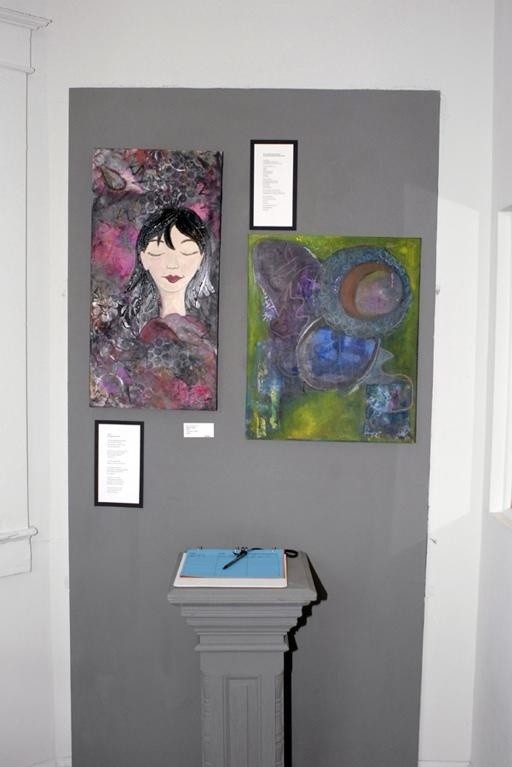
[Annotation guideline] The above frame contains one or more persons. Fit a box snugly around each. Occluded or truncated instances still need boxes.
[114,204,217,402]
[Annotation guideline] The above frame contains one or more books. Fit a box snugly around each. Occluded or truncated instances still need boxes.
[179,544,285,581]
[173,550,288,589]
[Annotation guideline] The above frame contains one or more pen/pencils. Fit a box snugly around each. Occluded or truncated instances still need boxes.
[223,551,247,569]
